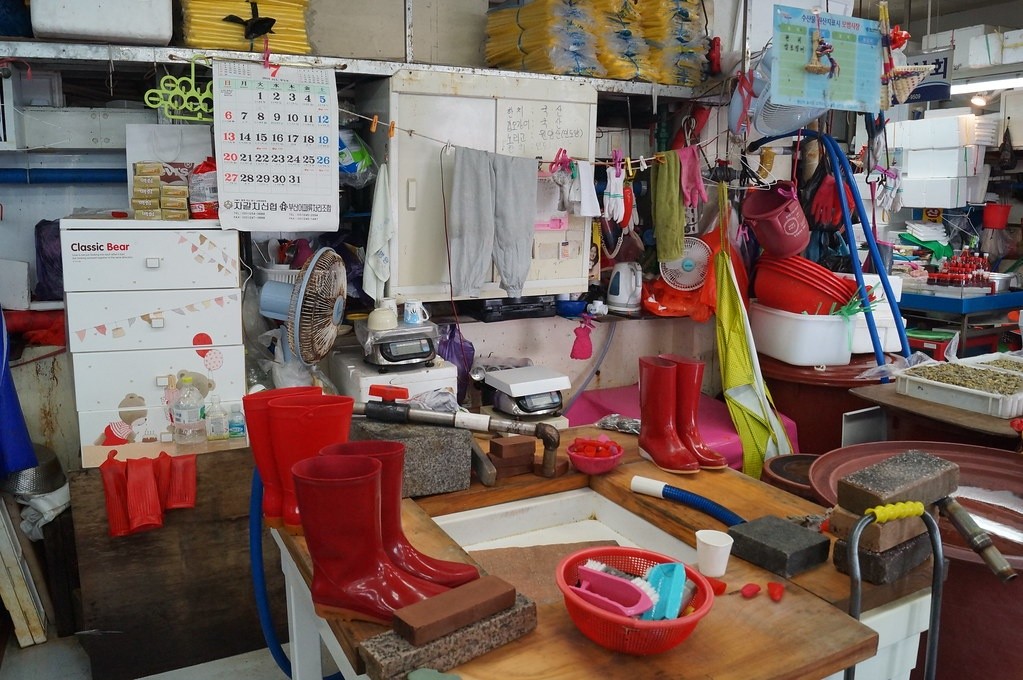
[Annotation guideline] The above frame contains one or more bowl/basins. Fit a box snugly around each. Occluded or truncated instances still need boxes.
[368,307,397,330]
[755,250,854,315]
[555,300,587,317]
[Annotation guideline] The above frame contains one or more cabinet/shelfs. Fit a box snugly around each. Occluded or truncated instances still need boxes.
[356,71,599,305]
[997,89,1023,147]
[0,59,176,152]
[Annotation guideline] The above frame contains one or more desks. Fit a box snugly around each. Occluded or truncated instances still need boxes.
[849,382,1023,442]
[266,425,948,680]
[896,292,1023,359]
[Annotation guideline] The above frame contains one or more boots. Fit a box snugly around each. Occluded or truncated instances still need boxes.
[266,393,354,535]
[659,354,728,470]
[242,386,325,530]
[638,356,699,473]
[320,439,479,587]
[293,455,450,627]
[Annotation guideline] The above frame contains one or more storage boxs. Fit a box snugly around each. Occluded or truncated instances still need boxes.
[30,0,174,48]
[59,163,250,466]
[327,348,459,404]
[749,266,1022,421]
[846,102,987,250]
[920,23,1023,69]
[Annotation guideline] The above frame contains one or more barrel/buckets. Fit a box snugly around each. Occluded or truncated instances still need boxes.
[742,180,810,259]
[983,203,1013,230]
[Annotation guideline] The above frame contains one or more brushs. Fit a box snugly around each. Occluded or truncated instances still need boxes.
[568,558,660,616]
[637,562,686,620]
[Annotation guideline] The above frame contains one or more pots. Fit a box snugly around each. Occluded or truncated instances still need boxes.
[989,273,1019,293]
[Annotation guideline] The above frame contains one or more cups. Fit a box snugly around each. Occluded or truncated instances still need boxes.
[404,299,429,325]
[380,298,398,317]
[695,530,734,577]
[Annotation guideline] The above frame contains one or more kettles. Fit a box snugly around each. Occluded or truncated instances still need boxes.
[605,261,642,312]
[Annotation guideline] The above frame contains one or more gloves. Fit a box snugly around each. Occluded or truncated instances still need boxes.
[609,169,625,223]
[675,143,708,207]
[99,448,197,538]
[623,181,639,234]
[811,174,855,224]
[603,169,613,222]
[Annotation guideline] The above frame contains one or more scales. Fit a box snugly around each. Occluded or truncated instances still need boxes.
[354,317,441,374]
[484,365,572,421]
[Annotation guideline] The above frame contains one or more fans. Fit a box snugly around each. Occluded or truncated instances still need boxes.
[728,47,830,138]
[258,247,348,395]
[659,236,713,292]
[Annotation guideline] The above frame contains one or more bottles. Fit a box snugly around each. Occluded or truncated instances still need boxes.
[926,246,990,288]
[229,404,246,438]
[173,376,206,444]
[205,395,230,441]
[990,281,996,294]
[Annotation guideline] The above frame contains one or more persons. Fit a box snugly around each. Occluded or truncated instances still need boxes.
[591,241,600,274]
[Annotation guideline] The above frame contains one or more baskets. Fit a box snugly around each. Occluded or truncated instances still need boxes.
[558,546,715,656]
[565,440,624,475]
